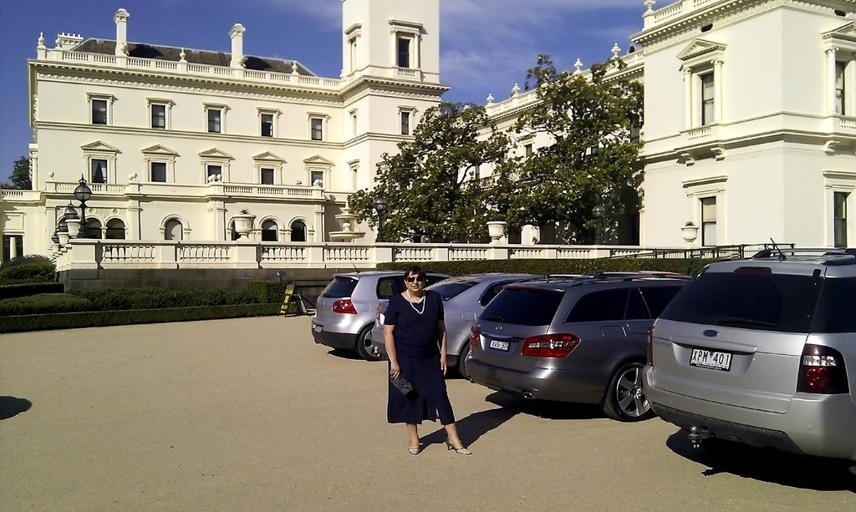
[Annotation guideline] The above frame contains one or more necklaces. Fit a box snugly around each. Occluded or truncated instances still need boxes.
[406,289,426,316]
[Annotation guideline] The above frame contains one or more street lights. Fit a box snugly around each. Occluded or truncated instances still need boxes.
[50,233,58,243]
[57,217,67,231]
[73,173,96,238]
[63,200,80,219]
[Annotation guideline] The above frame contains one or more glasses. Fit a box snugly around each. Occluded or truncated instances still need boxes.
[407,276,424,282]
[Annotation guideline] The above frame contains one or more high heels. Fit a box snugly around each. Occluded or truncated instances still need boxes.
[446,439,472,455]
[408,435,420,455]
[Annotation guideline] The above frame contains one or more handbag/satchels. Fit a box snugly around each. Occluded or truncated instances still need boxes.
[390,373,413,396]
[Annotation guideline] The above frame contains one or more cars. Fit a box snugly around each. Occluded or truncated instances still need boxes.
[311,270,453,361]
[370,270,551,380]
[641,248,856,465]
[464,269,691,423]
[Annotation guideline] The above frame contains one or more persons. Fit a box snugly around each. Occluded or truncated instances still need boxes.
[381,265,472,455]
